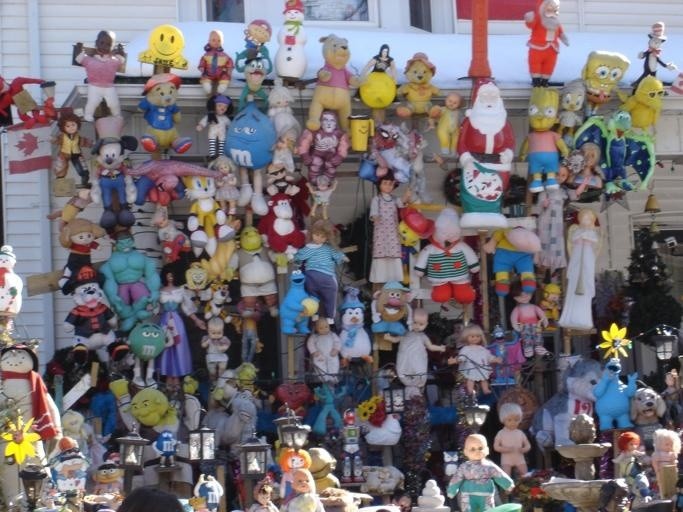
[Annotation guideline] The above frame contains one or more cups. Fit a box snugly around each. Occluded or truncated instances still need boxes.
[347,114,375,153]
